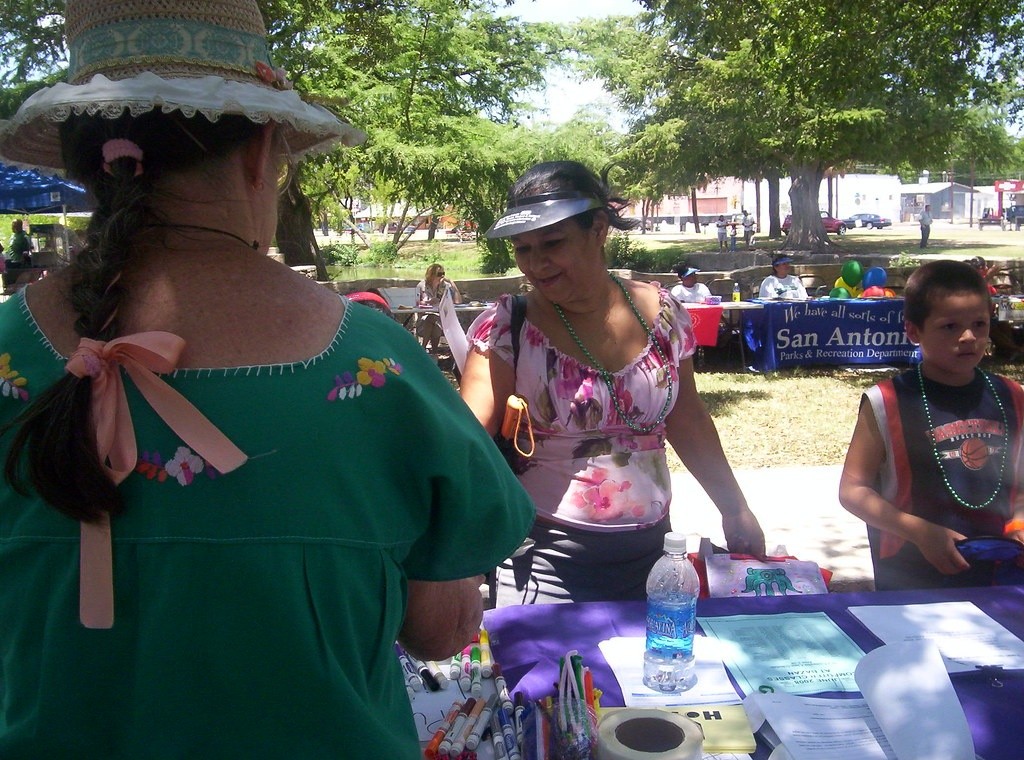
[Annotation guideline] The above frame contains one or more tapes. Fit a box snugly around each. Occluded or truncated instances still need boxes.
[597,707,702,760]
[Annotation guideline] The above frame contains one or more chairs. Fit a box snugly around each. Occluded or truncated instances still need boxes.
[413,312,443,340]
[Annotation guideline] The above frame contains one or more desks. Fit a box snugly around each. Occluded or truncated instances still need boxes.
[483,584,1023,760]
[396,621,516,760]
[370,303,493,313]
[6,266,61,281]
[681,301,765,374]
[738,295,923,377]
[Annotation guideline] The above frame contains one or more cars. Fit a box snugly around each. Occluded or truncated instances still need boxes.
[841,213,892,229]
[342,224,370,234]
[982,204,1024,227]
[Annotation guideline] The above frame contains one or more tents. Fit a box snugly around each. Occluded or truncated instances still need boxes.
[0,164,101,274]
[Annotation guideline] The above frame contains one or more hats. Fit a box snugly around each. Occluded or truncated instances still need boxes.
[771,257,794,265]
[74,229,85,236]
[342,291,392,314]
[482,190,604,240]
[6,0,364,180]
[681,266,697,278]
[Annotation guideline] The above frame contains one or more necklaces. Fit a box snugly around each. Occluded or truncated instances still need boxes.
[550,269,674,434]
[130,221,263,252]
[917,360,1010,511]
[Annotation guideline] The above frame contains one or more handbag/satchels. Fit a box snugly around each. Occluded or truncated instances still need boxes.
[685,538,834,600]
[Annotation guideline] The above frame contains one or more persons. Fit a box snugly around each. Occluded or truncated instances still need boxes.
[758,254,809,301]
[838,259,1024,592]
[67,228,99,269]
[0,218,31,295]
[962,256,1024,362]
[741,210,756,251]
[670,261,731,365]
[918,204,934,250]
[728,224,738,253]
[715,214,731,255]
[0,0,539,760]
[457,159,768,609]
[415,263,463,359]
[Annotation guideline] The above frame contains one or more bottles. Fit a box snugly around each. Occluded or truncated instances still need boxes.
[732,283,740,302]
[643,532,700,693]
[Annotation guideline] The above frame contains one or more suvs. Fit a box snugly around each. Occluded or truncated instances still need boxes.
[783,210,846,234]
[379,221,416,235]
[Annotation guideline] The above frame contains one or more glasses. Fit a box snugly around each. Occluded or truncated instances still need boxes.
[436,271,446,277]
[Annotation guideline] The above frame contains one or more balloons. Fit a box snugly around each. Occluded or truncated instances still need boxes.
[828,259,897,299]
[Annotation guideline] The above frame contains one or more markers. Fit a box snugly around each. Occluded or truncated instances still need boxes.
[515,691,526,745]
[450,644,471,691]
[479,630,492,679]
[471,647,481,695]
[395,641,421,702]
[439,697,486,756]
[407,653,439,692]
[423,661,449,690]
[490,707,520,760]
[425,700,461,760]
[491,664,514,715]
[467,694,499,750]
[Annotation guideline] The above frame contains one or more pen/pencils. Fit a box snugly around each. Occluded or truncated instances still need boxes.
[534,697,555,721]
[583,667,594,707]
